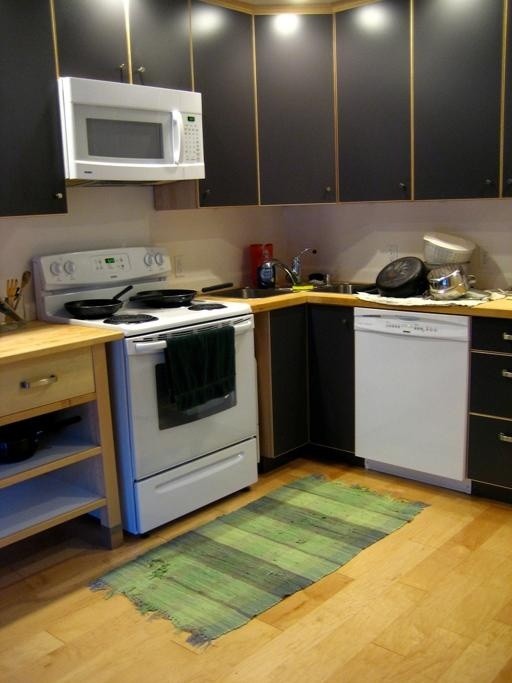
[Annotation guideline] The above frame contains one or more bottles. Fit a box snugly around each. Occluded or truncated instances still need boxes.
[338,282,353,294]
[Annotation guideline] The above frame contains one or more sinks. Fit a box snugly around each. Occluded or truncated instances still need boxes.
[310,283,377,294]
[198,286,297,300]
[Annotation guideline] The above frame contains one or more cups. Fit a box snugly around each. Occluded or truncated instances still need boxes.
[0,293,25,333]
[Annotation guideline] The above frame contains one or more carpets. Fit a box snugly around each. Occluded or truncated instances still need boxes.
[87,474,430,646]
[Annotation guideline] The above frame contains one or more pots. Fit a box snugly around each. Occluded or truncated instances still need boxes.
[353,257,478,301]
[1,414,83,465]
[64,283,233,320]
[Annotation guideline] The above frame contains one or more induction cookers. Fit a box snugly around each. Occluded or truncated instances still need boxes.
[33,246,252,337]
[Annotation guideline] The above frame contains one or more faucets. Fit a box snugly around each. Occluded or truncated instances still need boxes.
[261,259,299,284]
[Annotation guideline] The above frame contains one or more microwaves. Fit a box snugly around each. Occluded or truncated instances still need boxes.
[57,75,205,182]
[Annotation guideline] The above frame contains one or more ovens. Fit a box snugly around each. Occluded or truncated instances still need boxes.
[122,315,261,536]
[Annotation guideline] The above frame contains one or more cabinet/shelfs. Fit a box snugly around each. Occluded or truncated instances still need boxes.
[256,302,361,475]
[0,319,125,552]
[411,0,505,200]
[334,1,413,204]
[0,0,67,215]
[52,0,193,90]
[253,8,337,209]
[468,317,510,505]
[191,0,261,211]
